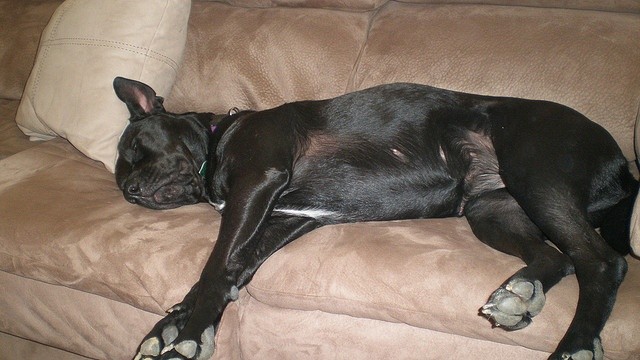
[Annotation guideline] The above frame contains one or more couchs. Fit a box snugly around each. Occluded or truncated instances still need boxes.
[0,2,640,359]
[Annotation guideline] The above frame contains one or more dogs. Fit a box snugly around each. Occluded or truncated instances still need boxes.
[112,77,639,360]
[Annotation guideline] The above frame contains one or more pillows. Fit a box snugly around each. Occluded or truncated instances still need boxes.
[15,0,192,174]
